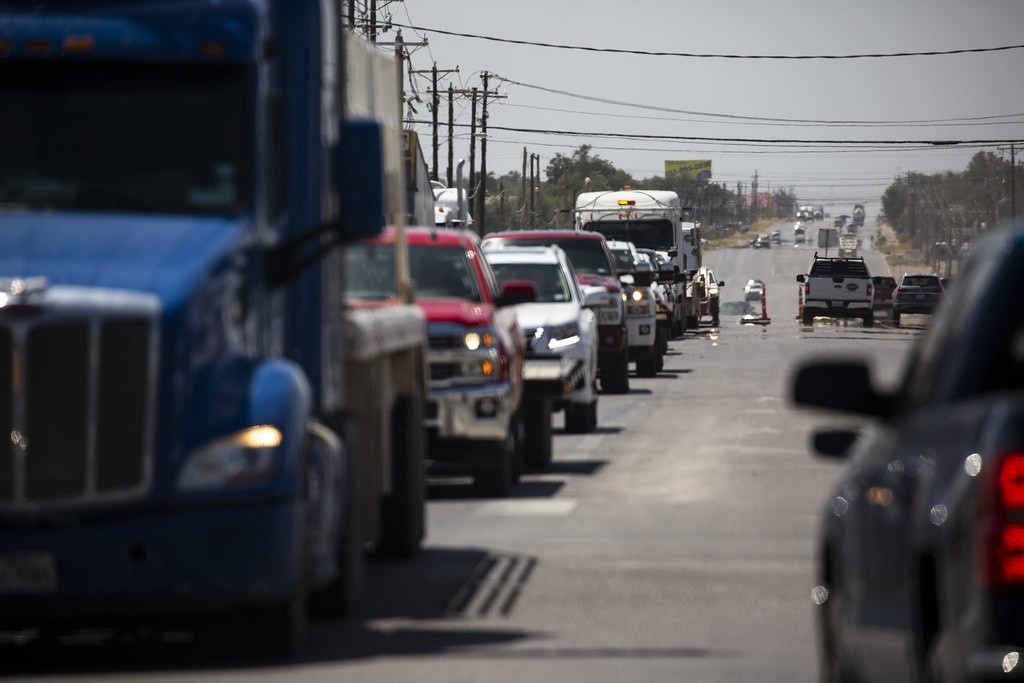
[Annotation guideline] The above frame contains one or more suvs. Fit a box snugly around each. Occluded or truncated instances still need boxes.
[480,242,611,432]
[872,272,951,320]
[606,238,665,379]
[635,249,687,356]
[339,226,536,500]
[483,232,654,394]
[796,251,881,328]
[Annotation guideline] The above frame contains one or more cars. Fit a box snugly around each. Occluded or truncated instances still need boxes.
[795,223,805,243]
[708,269,725,315]
[834,217,856,232]
[786,216,1024,683]
[796,206,824,222]
[742,279,766,301]
[752,227,782,248]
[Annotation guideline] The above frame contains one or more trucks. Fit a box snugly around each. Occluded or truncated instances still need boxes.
[575,189,708,332]
[853,204,866,225]
[0,0,432,657]
